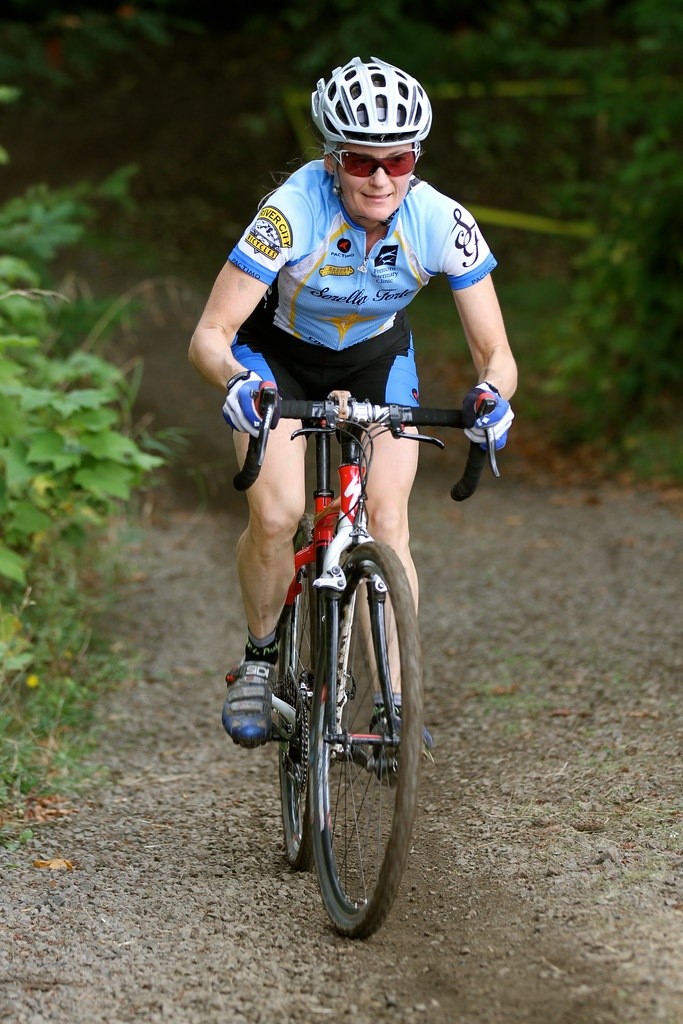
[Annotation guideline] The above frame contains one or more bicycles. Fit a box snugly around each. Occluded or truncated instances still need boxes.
[220,379,502,944]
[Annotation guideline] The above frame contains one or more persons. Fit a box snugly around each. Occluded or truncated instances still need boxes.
[188,54,516,788]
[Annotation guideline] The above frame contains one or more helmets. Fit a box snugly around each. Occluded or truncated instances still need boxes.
[310,54,432,146]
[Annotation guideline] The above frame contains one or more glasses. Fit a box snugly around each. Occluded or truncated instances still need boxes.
[325,143,420,177]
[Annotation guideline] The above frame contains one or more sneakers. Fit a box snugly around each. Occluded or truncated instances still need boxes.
[376,720,433,788]
[222,656,276,743]
